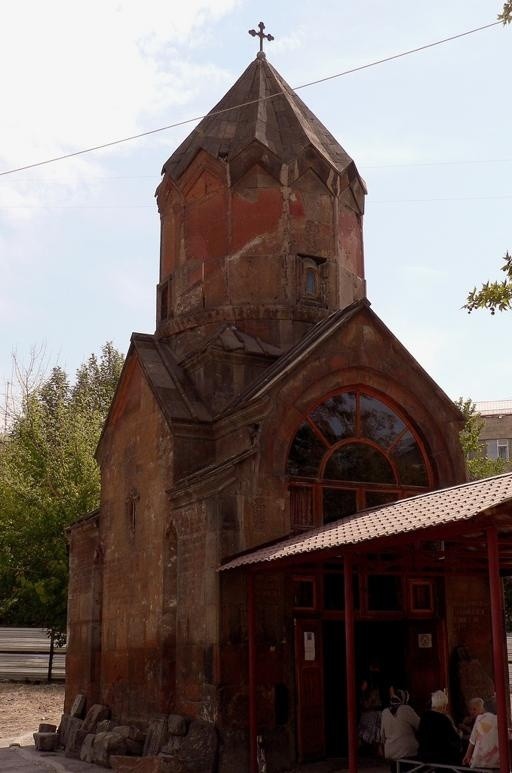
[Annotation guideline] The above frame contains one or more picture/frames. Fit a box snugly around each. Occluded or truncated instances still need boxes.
[293,576,317,610]
[410,582,434,612]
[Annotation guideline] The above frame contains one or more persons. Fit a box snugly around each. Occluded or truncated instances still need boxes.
[452,697,485,735]
[414,689,465,773]
[379,689,422,772]
[461,697,502,773]
[357,678,385,765]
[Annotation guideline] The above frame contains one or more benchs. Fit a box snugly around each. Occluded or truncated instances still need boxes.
[394,756,499,773]
